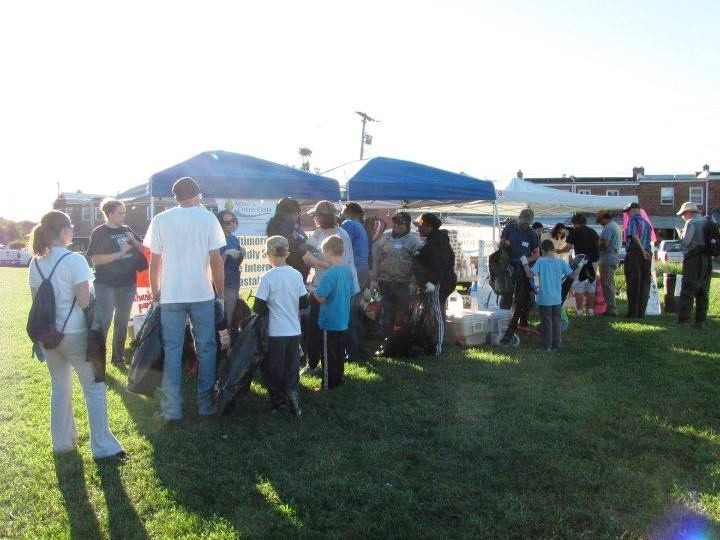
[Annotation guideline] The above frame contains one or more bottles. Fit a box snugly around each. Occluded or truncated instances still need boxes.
[470,296,478,316]
[447,289,463,318]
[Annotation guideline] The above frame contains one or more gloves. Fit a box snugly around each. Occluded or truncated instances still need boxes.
[224,247,246,259]
[214,295,224,318]
[425,281,435,294]
[577,258,588,268]
[519,255,528,265]
[152,301,159,310]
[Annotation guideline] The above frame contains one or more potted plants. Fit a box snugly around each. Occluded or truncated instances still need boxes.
[657,260,684,296]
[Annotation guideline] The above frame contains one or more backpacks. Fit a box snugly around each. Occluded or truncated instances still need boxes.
[26,251,80,349]
[489,240,513,295]
[702,215,720,256]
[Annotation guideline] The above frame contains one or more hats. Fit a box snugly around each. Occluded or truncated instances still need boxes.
[172,177,200,201]
[595,209,609,223]
[623,202,640,212]
[305,201,342,216]
[391,213,411,225]
[676,202,697,215]
[264,235,288,256]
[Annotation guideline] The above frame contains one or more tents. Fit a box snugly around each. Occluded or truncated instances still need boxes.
[116,149,638,242]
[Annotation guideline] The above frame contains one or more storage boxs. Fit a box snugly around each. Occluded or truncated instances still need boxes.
[451,320,488,346]
[490,319,516,347]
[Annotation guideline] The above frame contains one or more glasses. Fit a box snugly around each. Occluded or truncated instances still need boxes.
[222,218,236,226]
[392,221,409,226]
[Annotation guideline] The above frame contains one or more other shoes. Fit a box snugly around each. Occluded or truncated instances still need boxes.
[691,319,705,329]
[287,390,301,418]
[679,322,690,328]
[301,367,322,376]
[600,312,616,316]
[314,387,334,393]
[265,400,287,417]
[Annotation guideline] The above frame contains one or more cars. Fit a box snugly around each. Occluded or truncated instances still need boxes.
[657,240,685,264]
[618,240,630,263]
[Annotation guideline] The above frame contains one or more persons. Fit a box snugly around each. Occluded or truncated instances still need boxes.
[499,208,541,345]
[624,201,654,317]
[540,223,567,259]
[300,200,360,377]
[340,201,371,291]
[86,200,148,367]
[372,211,421,357]
[314,235,355,393]
[253,235,309,417]
[596,210,622,316]
[216,211,246,324]
[143,176,227,421]
[266,196,312,283]
[414,213,451,356]
[556,215,600,317]
[532,222,544,235]
[674,202,713,328]
[521,239,589,350]
[27,209,126,460]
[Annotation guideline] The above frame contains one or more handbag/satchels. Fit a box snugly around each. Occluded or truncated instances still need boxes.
[135,248,149,272]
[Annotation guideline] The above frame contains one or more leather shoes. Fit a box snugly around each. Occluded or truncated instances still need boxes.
[94,451,126,462]
